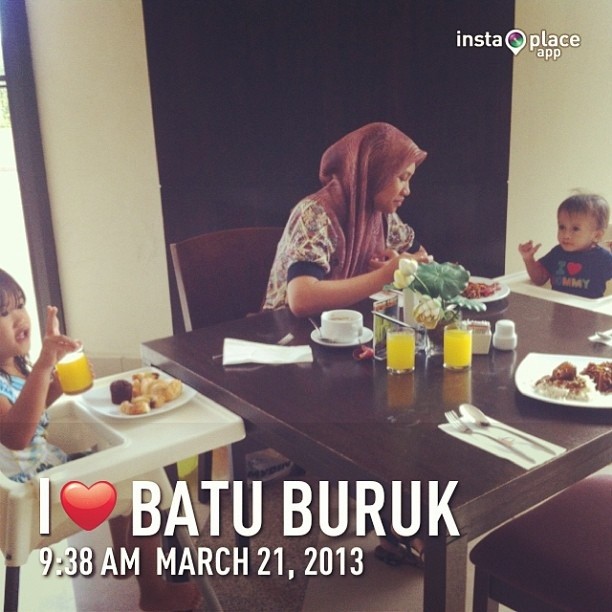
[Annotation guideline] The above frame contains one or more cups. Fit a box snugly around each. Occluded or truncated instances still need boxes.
[321,310,364,343]
[385,326,415,374]
[50,342,93,395]
[443,324,472,372]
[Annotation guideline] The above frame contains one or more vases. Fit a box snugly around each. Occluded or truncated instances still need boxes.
[421,311,463,369]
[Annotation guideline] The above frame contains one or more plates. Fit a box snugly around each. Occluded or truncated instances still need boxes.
[310,326,373,347]
[86,380,197,420]
[515,352,612,408]
[460,275,510,303]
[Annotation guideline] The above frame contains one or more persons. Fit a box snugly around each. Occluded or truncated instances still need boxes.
[0,266,202,612]
[517,192,612,304]
[260,121,438,321]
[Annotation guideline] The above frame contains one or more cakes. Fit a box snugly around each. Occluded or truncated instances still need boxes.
[110,379,133,405]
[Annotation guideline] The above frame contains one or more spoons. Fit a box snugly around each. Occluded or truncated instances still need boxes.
[308,317,335,343]
[458,404,556,455]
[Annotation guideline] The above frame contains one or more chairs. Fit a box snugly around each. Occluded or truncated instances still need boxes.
[469,474,612,612]
[0,366,246,612]
[170,228,304,571]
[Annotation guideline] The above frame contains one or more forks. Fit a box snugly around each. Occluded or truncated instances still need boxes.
[212,333,293,359]
[444,409,536,465]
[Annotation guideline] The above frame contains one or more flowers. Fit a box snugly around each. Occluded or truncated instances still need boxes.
[383,255,487,345]
